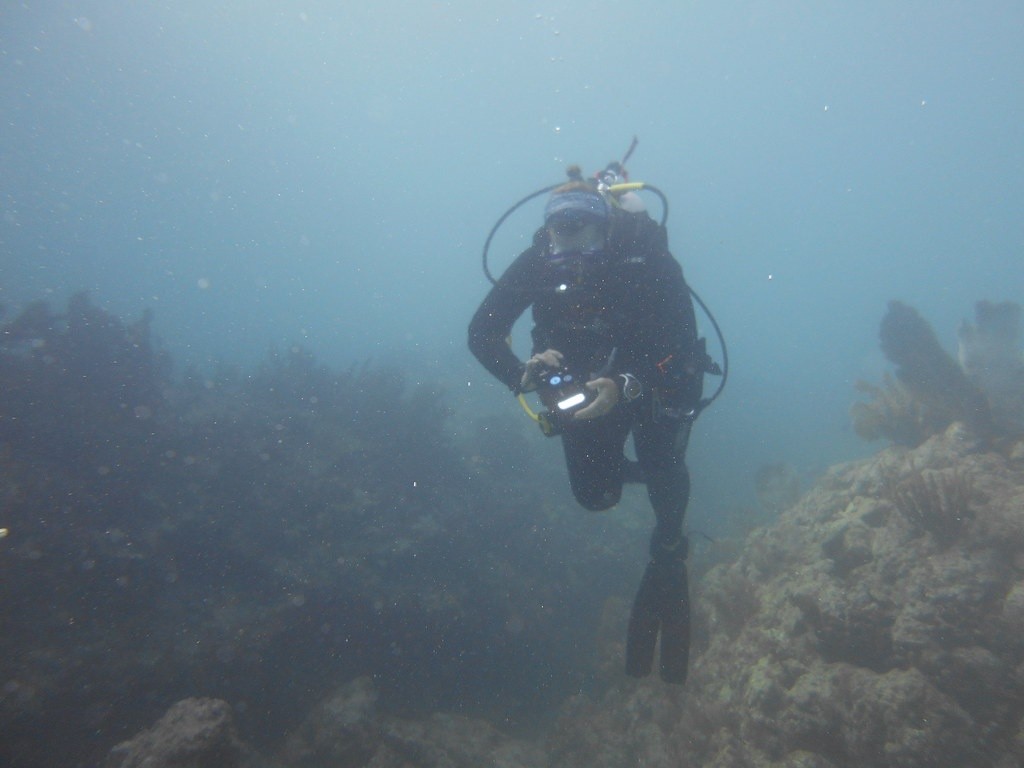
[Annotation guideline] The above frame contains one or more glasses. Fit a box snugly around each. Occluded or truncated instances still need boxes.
[544,249,606,274]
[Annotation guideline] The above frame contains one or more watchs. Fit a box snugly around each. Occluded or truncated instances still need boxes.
[619,373,642,409]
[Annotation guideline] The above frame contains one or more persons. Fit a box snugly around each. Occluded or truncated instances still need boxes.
[469,179,706,682]
